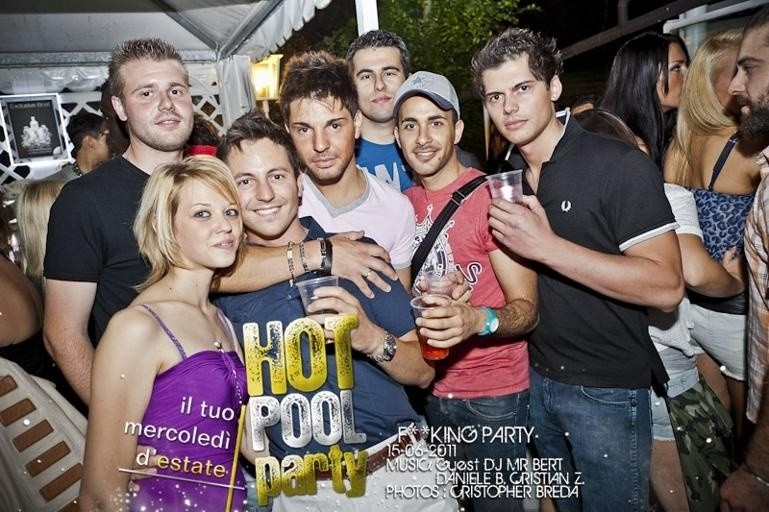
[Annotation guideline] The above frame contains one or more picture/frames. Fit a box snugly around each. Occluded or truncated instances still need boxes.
[0,92,73,168]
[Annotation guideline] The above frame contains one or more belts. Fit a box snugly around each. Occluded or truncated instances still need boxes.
[274,423,423,487]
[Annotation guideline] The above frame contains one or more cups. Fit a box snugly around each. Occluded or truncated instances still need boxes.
[411,268,460,360]
[295,275,339,344]
[485,169,526,207]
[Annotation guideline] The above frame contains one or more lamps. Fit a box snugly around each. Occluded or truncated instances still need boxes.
[249,48,285,118]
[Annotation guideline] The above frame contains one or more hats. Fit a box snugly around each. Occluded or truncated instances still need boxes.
[391,68,462,120]
